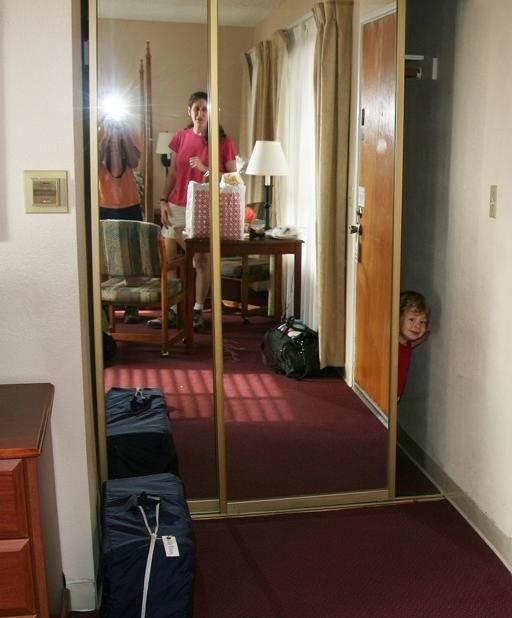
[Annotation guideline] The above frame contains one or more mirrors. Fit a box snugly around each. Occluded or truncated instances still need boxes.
[90,0,400,516]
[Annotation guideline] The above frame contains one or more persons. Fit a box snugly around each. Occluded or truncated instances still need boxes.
[146,92,237,332]
[397,291,430,406]
[97,92,144,324]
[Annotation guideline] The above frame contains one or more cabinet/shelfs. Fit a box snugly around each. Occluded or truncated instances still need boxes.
[0,384,71,618]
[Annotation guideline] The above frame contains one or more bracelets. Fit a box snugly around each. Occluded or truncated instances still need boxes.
[160,199,168,203]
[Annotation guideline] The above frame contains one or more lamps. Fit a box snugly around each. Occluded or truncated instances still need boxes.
[245,140,291,230]
[156,132,176,168]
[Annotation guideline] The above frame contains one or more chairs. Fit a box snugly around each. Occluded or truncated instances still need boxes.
[220,256,270,316]
[100,219,190,357]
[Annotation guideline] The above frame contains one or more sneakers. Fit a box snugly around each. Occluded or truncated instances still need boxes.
[147,309,176,329]
[193,309,204,329]
[124,306,139,323]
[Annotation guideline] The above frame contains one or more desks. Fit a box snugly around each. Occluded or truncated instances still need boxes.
[175,224,305,348]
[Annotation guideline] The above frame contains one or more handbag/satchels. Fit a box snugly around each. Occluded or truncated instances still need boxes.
[101,474,198,618]
[269,316,328,378]
[105,386,178,480]
